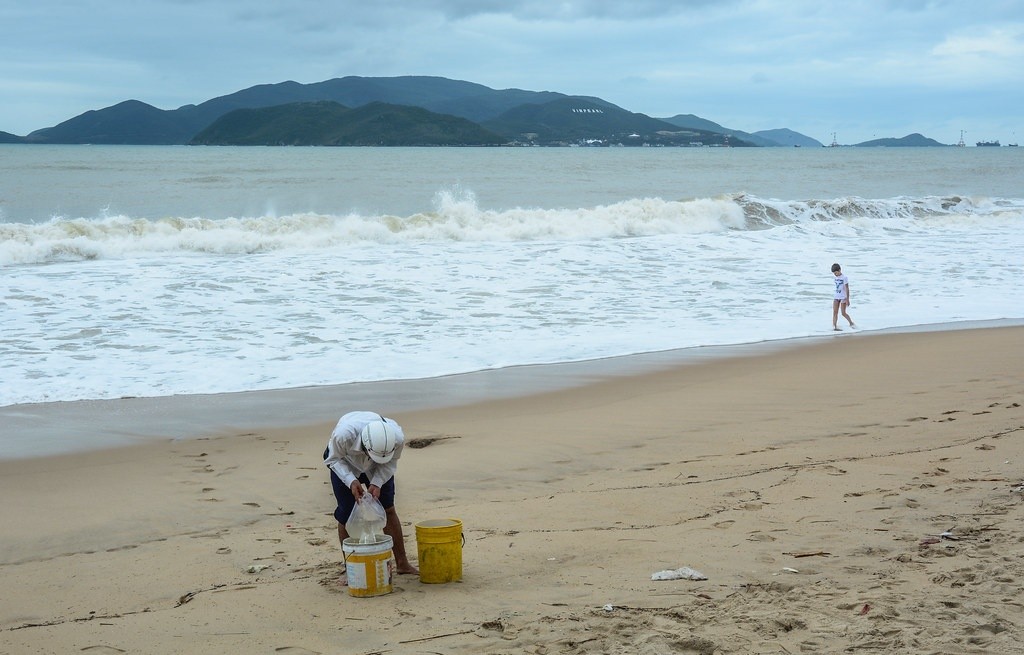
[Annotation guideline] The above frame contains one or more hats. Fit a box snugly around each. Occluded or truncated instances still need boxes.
[362,421,395,464]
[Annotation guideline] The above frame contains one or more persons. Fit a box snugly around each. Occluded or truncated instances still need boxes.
[831,263,855,331]
[322,410,420,586]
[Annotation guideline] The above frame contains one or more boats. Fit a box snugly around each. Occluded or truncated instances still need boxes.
[976,140,1000,146]
[1009,144,1018,146]
[709,137,731,147]
[794,145,801,147]
[690,142,703,146]
[822,132,852,147]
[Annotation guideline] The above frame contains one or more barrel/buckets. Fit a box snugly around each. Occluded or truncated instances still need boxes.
[342,533,394,598]
[415,519,465,584]
